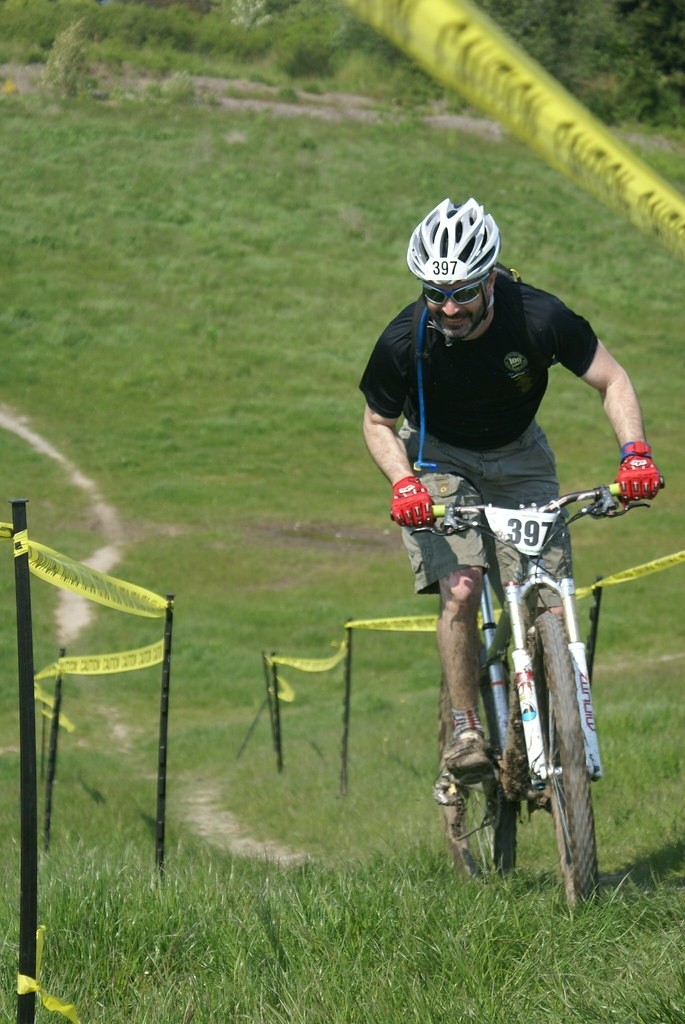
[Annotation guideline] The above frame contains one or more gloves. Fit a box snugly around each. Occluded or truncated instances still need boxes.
[391,477,438,528]
[613,441,660,508]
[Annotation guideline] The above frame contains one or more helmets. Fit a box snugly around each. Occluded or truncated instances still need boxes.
[405,197,501,285]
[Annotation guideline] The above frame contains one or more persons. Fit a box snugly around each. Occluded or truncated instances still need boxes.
[358,197,660,811]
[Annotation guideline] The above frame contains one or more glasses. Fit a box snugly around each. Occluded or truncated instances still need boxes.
[420,273,493,304]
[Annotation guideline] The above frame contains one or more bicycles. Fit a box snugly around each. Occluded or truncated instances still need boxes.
[390,472,668,925]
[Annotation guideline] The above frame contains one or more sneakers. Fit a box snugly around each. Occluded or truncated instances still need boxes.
[443,729,491,778]
